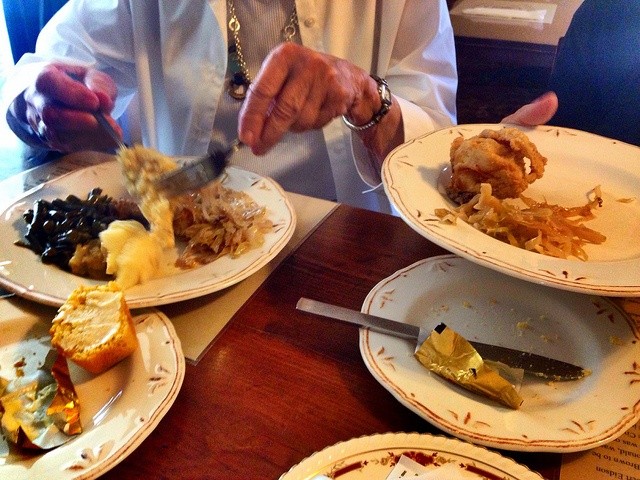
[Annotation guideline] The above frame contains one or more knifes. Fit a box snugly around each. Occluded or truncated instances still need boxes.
[295,294,592,380]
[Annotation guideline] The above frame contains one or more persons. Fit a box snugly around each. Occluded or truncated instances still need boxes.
[498,0,640,147]
[5,1,459,217]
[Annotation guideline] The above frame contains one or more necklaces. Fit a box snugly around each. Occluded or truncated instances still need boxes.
[227,1,297,99]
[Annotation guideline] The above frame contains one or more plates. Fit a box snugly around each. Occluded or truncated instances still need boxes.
[0,294,187,480]
[276,431,543,480]
[358,254,640,454]
[0,157,297,310]
[381,122,640,297]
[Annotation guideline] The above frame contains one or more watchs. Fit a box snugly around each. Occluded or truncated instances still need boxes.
[342,75,392,132]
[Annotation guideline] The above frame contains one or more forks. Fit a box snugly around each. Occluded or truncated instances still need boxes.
[118,139,247,201]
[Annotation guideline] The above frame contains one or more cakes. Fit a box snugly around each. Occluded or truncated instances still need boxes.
[48,281,136,375]
